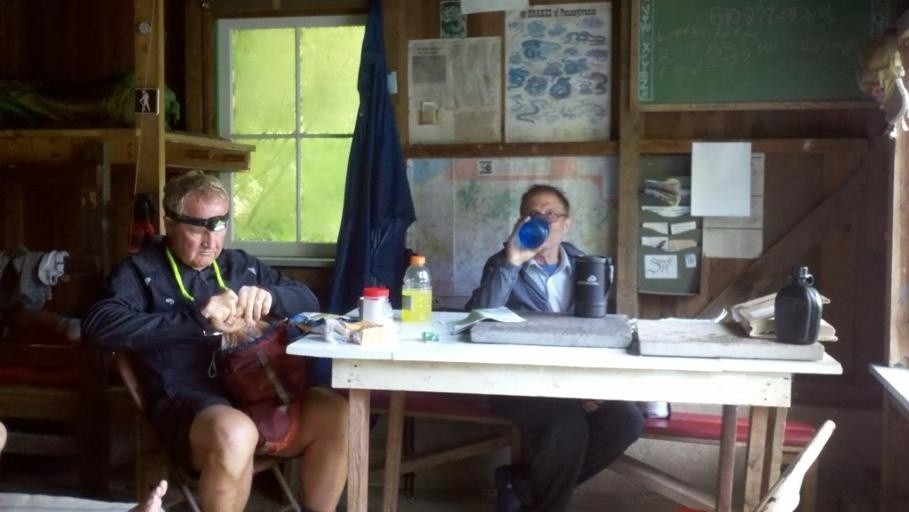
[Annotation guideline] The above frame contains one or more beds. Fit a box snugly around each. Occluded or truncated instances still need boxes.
[1,128,253,470]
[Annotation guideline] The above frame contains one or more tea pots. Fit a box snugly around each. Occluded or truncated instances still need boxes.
[574,256,614,317]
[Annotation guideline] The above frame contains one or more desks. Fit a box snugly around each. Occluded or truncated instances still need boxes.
[285,308,844,512]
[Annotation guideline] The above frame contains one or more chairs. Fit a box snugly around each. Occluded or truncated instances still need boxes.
[112,351,301,512]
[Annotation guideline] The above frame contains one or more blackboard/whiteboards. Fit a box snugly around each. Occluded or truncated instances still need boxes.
[630,2,901,110]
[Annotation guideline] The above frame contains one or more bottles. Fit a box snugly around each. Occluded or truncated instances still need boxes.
[401,255,436,324]
[357,287,394,348]
[519,218,552,246]
[775,266,821,345]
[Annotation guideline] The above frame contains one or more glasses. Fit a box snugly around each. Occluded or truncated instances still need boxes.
[521,207,567,225]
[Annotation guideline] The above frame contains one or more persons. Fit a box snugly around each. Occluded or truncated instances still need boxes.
[464,184,646,512]
[128,479,167,512]
[81,170,347,512]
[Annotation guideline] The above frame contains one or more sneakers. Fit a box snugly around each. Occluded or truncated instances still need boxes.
[493,463,524,512]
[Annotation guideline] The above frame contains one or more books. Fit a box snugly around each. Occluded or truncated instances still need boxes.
[732,291,837,341]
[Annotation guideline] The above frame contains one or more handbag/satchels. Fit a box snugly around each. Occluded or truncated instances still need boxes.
[206,319,311,462]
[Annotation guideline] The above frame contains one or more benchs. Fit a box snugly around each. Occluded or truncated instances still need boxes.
[337,389,815,512]
